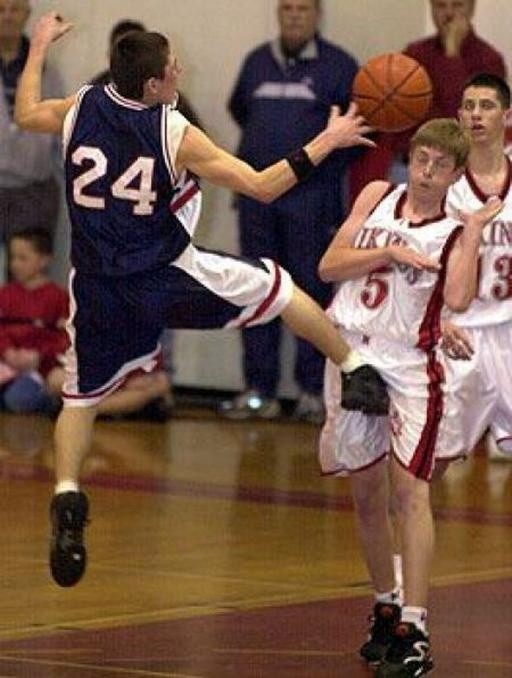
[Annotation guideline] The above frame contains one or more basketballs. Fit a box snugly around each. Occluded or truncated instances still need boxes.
[351,54,433,132]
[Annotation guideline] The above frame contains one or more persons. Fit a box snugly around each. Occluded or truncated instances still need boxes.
[16,10,390,588]
[311,116,504,677]
[0,1,61,273]
[429,67,512,486]
[42,294,178,424]
[346,1,507,209]
[0,224,71,417]
[82,18,196,422]
[216,1,361,426]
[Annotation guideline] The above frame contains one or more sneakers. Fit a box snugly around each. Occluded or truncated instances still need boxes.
[341,363,390,416]
[50,490,89,586]
[360,602,432,673]
[121,397,173,424]
[296,395,325,425]
[218,393,283,420]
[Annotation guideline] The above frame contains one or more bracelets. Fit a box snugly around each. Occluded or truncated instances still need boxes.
[287,147,316,185]
